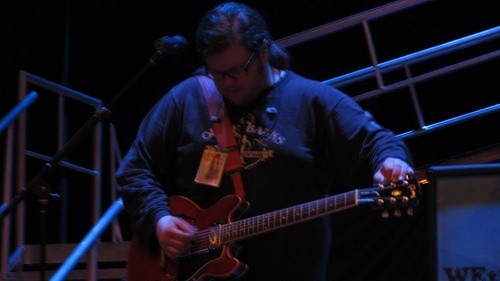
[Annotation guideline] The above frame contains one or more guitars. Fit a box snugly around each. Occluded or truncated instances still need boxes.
[127,169,430,281]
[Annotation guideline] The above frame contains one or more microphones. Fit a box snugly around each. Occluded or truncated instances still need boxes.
[155,34,188,55]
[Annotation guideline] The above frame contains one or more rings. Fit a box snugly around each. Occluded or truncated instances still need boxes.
[162,247,165,252]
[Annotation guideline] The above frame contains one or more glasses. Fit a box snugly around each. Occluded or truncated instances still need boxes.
[204,49,258,81]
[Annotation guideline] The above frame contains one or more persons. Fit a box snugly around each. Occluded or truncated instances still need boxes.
[110,0,419,281]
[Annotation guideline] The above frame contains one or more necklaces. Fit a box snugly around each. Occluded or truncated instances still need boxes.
[217,69,283,140]
